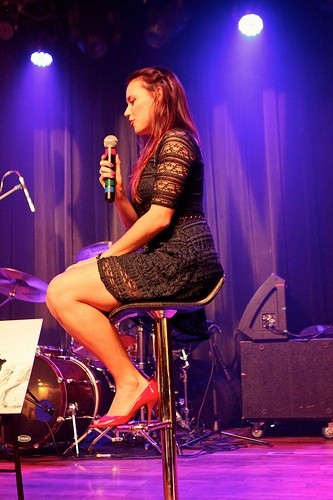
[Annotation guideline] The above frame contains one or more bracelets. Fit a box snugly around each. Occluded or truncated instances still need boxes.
[95,253,103,260]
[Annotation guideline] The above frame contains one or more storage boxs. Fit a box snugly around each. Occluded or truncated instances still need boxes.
[240,338,333,439]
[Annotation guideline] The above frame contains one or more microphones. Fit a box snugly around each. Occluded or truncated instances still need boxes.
[15,171,35,212]
[103,135,120,202]
[43,406,55,415]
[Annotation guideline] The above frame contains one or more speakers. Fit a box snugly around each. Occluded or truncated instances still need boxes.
[239,338,333,420]
[237,273,288,341]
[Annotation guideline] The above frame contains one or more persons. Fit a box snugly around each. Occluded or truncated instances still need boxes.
[46,68,225,428]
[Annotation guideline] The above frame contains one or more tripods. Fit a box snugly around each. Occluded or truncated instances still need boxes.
[61,318,271,458]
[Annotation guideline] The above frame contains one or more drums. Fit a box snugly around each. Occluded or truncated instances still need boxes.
[37,344,66,359]
[0,355,99,456]
[69,317,139,362]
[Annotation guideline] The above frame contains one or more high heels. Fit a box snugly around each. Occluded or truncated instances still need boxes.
[89,379,159,428]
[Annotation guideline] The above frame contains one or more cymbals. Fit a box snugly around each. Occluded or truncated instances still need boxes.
[0,267,49,303]
[74,241,114,262]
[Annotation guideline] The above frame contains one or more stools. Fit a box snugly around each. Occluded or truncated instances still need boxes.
[107,275,228,500]
